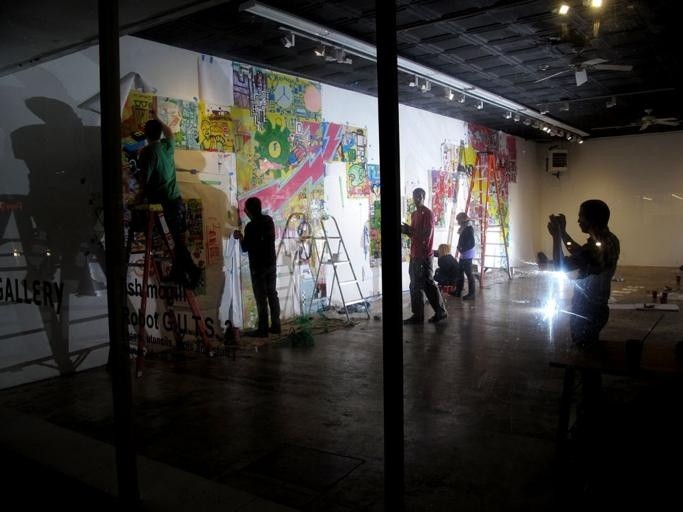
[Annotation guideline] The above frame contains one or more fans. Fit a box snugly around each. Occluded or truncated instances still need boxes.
[590,108,680,131]
[532,56,634,87]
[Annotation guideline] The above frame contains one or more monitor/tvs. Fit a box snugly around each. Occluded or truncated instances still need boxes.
[548,149,568,174]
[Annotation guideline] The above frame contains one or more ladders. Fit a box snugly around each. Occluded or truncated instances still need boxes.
[126,203,210,379]
[455,152,511,288]
[274,212,371,323]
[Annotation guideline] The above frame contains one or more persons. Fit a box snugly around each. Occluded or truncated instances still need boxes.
[233,197,282,338]
[400,188,448,325]
[450,213,476,300]
[129,101,189,278]
[432,244,462,290]
[546,199,620,441]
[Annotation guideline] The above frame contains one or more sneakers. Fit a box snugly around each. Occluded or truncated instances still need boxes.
[404,313,448,323]
[248,327,281,338]
[448,290,475,300]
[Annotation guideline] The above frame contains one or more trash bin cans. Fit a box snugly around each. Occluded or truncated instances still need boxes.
[166,350,203,397]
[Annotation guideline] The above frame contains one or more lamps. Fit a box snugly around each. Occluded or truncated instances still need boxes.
[538,96,617,116]
[279,31,585,145]
